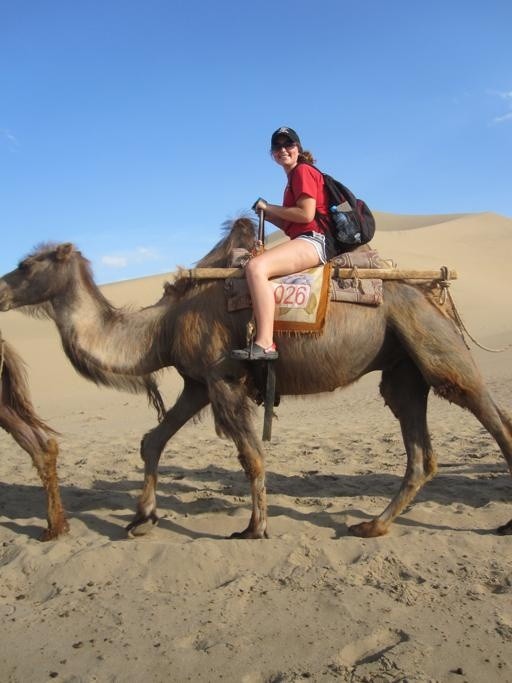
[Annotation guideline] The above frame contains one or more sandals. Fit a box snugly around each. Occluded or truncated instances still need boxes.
[230,342,279,362]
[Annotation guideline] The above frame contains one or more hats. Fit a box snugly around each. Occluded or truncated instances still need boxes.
[270,126,300,146]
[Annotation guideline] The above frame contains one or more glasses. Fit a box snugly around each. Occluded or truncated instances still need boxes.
[273,141,295,151]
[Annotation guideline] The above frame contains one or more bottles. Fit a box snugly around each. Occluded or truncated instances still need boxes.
[330,206,360,241]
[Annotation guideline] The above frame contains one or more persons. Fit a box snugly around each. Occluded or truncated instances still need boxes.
[231,126,376,361]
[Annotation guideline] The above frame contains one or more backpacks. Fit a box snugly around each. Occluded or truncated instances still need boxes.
[288,162,375,255]
